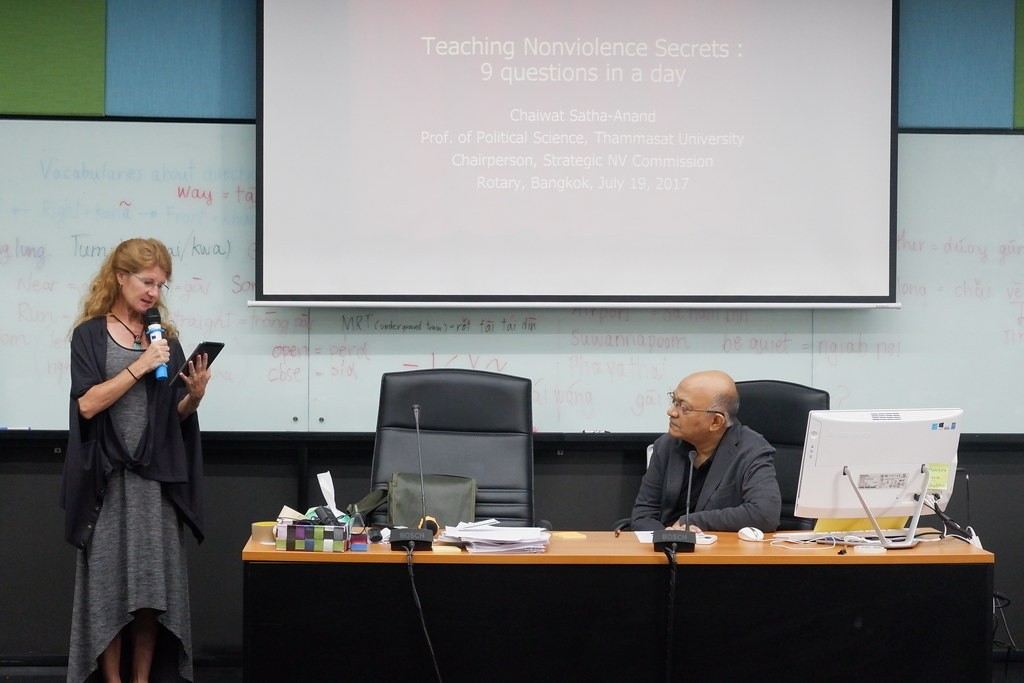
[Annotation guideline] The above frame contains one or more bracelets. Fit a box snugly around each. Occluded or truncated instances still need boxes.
[126,368,139,380]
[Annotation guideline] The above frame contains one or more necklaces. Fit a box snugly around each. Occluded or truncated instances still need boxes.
[109,310,145,349]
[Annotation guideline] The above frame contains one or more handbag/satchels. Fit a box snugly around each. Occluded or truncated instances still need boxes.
[387,472,478,527]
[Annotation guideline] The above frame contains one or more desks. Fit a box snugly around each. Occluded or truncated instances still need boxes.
[241,523,994,683]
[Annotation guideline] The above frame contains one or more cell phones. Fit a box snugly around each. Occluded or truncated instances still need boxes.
[696,535,717,545]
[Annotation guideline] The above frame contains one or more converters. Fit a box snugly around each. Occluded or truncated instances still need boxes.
[314,506,340,526]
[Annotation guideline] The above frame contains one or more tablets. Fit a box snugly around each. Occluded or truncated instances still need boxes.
[170,341,225,388]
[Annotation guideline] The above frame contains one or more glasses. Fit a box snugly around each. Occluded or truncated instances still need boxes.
[127,268,169,298]
[666,391,724,418]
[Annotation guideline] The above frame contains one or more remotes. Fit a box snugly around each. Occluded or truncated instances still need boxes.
[368,528,383,542]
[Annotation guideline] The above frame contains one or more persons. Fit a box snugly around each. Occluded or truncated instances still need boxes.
[64,238,210,683]
[632,371,782,532]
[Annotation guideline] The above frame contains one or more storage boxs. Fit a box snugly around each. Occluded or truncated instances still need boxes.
[275,519,351,552]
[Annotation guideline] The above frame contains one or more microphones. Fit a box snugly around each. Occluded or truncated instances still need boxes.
[143,307,168,380]
[653,451,698,552]
[390,404,433,550]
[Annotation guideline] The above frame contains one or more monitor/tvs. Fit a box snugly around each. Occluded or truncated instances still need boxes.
[794,408,962,548]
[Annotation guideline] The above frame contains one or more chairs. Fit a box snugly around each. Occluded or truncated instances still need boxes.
[370,367,536,527]
[734,379,831,531]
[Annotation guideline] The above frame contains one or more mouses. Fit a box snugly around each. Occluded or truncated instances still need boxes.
[738,527,764,541]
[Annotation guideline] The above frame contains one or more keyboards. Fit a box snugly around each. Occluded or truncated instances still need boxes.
[774,528,910,542]
